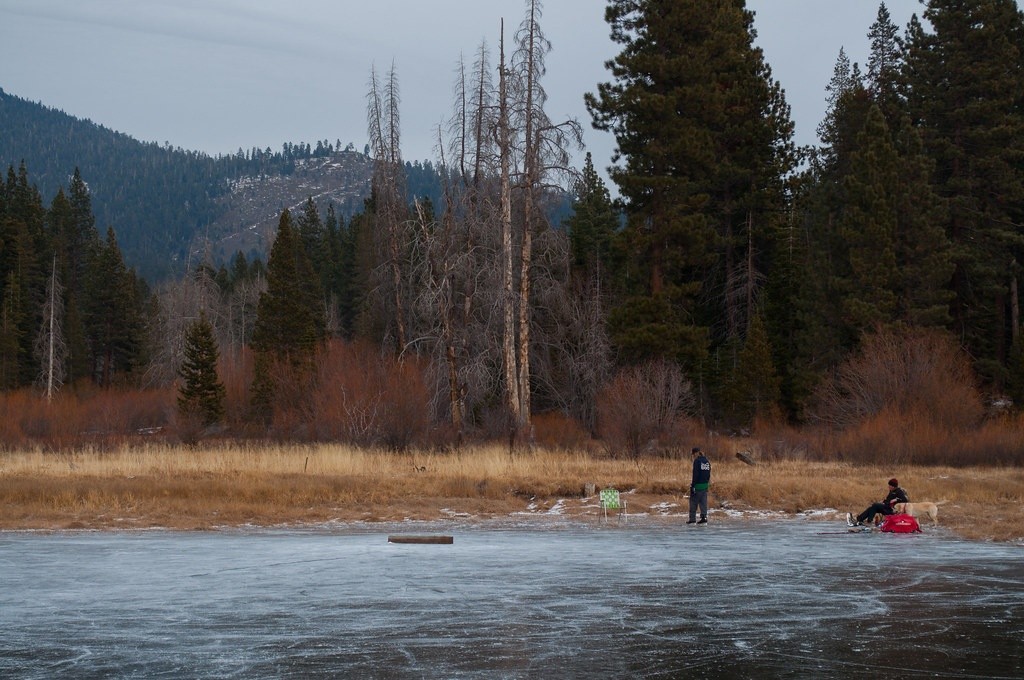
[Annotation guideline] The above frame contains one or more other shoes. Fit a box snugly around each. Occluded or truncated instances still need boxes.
[686,519,697,526]
[846,512,860,526]
[698,517,707,526]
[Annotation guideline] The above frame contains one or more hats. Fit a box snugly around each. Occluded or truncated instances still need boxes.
[691,448,701,455]
[888,478,898,487]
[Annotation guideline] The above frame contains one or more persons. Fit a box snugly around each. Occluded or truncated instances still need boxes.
[685,446,712,524]
[846,478,909,527]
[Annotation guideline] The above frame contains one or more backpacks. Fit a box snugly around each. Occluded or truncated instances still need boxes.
[881,514,922,533]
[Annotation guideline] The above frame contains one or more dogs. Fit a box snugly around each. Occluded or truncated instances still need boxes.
[892,502,938,529]
[874,513,885,527]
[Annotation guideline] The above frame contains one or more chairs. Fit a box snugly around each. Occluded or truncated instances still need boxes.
[598,489,628,525]
